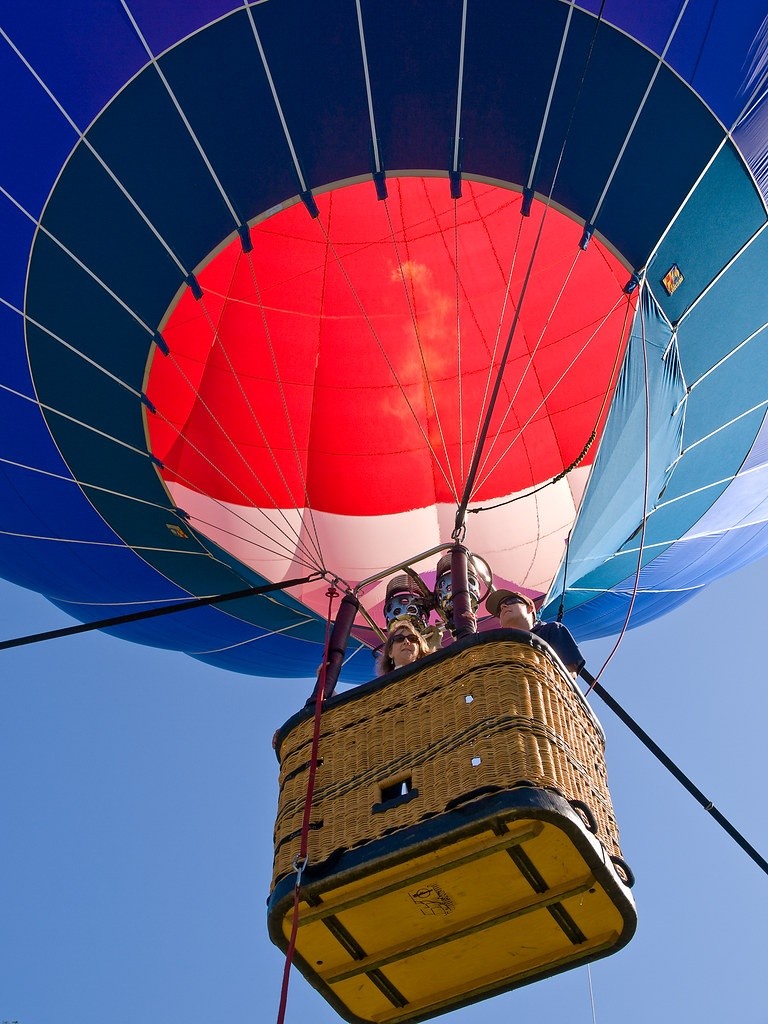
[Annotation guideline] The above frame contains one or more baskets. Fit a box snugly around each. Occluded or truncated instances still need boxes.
[265,627,640,1024]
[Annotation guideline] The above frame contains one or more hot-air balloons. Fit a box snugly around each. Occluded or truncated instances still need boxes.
[1,0,766,1022]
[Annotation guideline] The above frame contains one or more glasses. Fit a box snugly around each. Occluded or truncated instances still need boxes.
[497,596,524,605]
[389,634,418,643]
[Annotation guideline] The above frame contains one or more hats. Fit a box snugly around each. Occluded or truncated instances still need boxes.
[485,589,536,622]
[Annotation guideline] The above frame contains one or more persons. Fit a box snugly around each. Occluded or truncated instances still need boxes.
[486,588,586,680]
[385,620,429,670]
